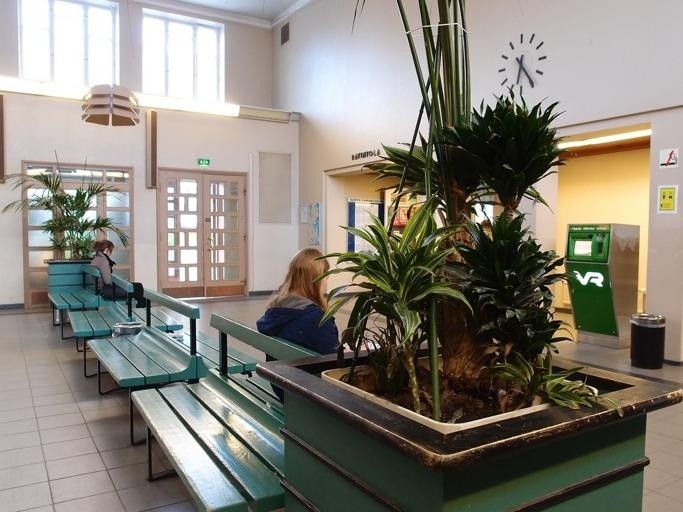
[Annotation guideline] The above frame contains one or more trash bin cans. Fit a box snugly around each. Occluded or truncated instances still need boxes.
[630,314,666,369]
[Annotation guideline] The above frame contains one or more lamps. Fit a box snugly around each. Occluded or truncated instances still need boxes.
[81,1,141,128]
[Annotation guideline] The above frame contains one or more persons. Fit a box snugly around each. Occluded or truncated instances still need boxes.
[255,246,345,404]
[90,238,146,309]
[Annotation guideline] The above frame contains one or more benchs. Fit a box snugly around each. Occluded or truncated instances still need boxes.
[44,265,323,512]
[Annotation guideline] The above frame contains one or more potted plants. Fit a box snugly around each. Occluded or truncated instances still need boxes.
[0,148,130,292]
[256,1,682,512]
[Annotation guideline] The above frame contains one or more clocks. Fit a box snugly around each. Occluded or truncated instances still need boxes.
[496,32,546,95]
